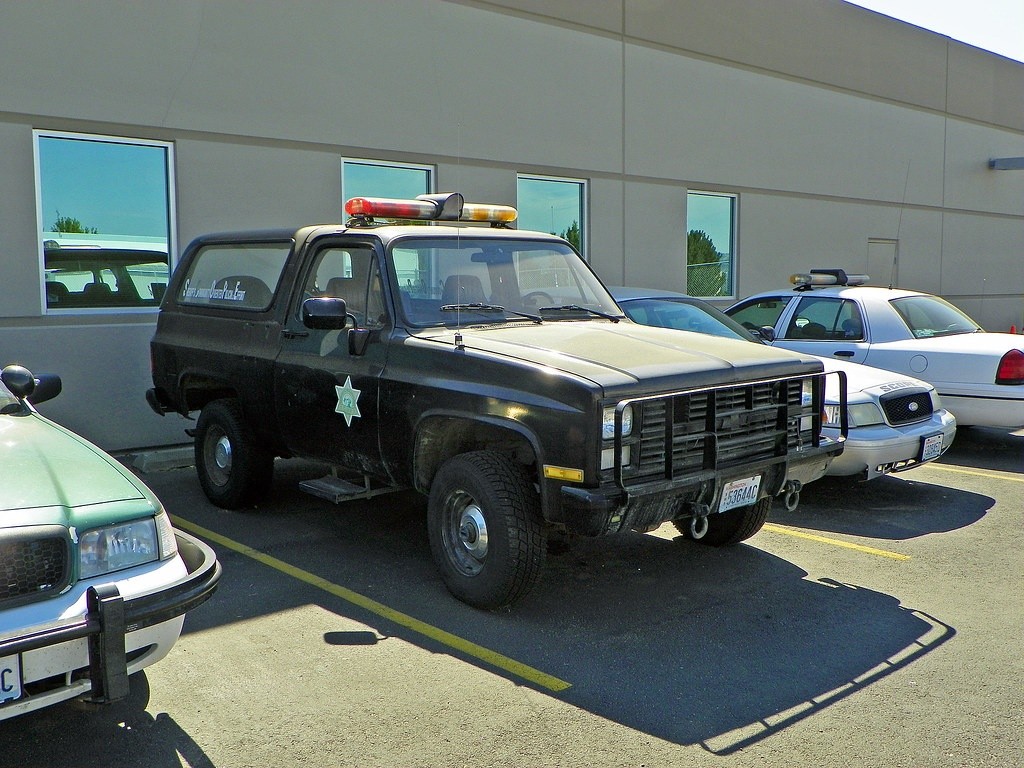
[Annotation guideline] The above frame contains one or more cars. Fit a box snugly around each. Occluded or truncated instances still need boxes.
[717,266,1024,438]
[0,364,223,731]
[510,281,958,497]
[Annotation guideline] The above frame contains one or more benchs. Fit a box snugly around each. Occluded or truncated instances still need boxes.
[45,282,111,296]
[209,276,368,312]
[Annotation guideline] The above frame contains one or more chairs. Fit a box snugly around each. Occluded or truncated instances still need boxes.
[442,275,488,320]
[835,319,861,339]
[373,290,416,324]
[800,323,826,339]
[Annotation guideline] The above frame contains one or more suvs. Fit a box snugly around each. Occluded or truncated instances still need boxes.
[144,188,848,617]
[42,230,172,310]
